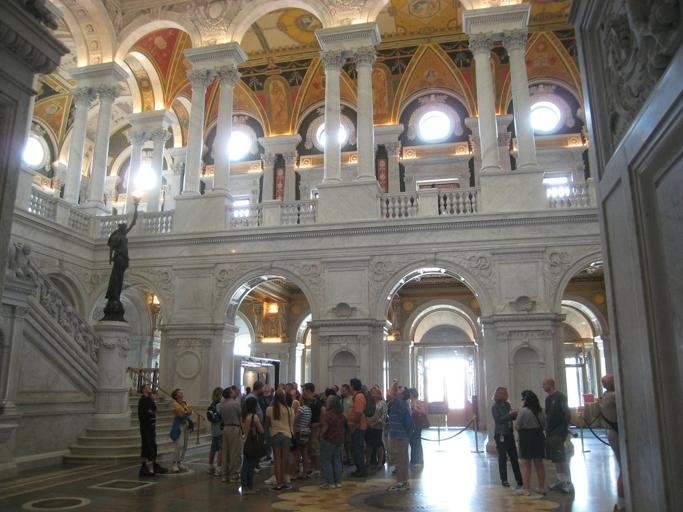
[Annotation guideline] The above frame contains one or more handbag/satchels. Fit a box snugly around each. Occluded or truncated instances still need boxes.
[415,414,429,428]
[289,438,298,452]
[243,432,260,457]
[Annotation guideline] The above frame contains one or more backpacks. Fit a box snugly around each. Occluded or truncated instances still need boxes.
[352,391,374,417]
[205,400,222,424]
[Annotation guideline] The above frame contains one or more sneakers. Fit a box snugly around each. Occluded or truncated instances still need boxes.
[153,464,168,473]
[178,464,186,472]
[206,456,423,496]
[171,464,179,473]
[502,480,572,497]
[139,466,155,477]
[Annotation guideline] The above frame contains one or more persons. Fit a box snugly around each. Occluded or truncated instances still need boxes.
[13,241,38,286]
[138,384,169,476]
[169,389,193,472]
[542,377,573,493]
[515,390,546,495]
[491,387,522,487]
[599,375,624,512]
[105,205,138,301]
[208,379,424,493]
